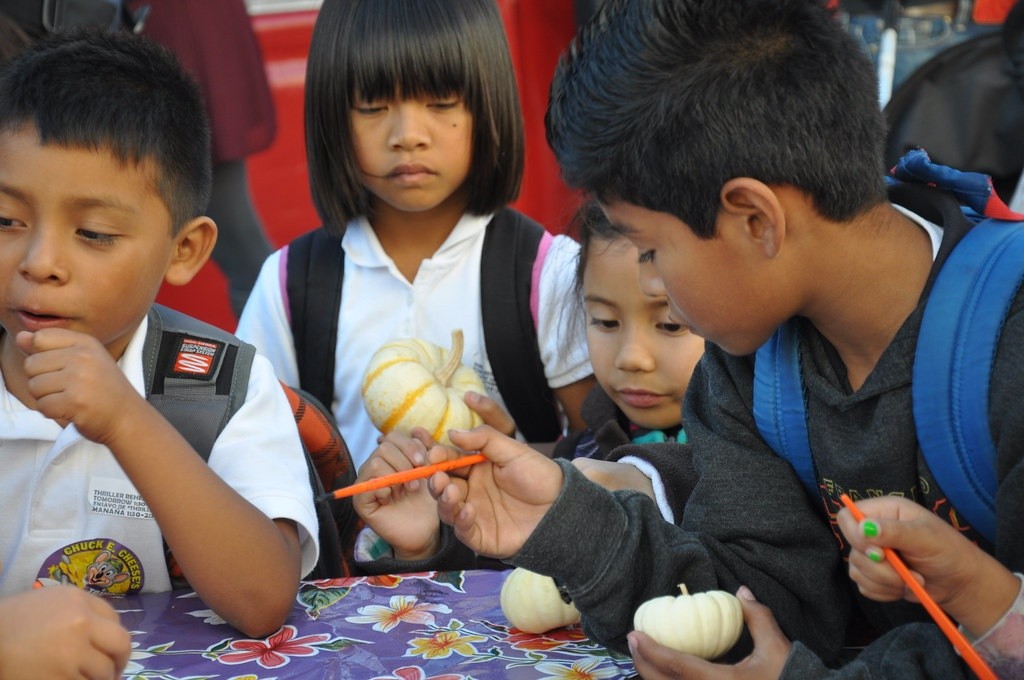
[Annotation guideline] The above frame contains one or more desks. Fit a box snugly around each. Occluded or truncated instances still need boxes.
[98,571,645,680]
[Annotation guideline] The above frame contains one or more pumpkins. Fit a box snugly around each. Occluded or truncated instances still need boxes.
[500,567,582,634]
[362,329,488,448]
[633,584,744,661]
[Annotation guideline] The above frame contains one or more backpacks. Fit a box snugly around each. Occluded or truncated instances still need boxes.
[143,302,358,592]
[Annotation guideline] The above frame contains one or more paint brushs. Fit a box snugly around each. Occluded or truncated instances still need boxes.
[317,455,491,506]
[822,478,1002,680]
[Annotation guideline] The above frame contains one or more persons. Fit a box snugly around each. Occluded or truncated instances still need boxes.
[1,0,319,679]
[836,496,1024,680]
[233,0,598,576]
[352,201,705,569]
[428,1,1024,680]
